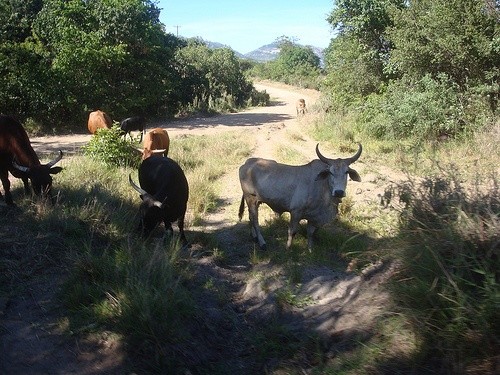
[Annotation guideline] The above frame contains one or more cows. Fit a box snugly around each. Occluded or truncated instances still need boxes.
[87,110,112,135]
[129,127,190,247]
[119,117,146,143]
[0,102,65,202]
[296,98,307,121]
[236,142,363,250]
